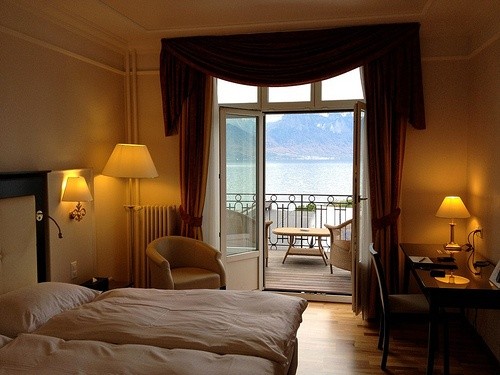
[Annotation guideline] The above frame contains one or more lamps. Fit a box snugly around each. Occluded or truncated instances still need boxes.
[435,195,471,251]
[61,176,93,222]
[102,144,158,287]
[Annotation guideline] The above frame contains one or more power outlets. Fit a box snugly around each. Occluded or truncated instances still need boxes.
[474,226,483,238]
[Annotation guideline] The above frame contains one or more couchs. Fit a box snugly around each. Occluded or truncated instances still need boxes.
[146,236,227,290]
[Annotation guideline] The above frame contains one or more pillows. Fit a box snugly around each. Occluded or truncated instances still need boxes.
[0,279,102,338]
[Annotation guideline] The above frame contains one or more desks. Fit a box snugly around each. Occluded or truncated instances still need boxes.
[400,243,500,375]
[271,227,330,266]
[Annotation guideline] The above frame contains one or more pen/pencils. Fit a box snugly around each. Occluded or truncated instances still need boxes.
[419,258,425,262]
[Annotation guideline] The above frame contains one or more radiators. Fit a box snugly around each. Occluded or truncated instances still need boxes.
[124,205,176,288]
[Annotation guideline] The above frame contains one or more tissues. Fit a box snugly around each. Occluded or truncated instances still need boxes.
[79,276,109,292]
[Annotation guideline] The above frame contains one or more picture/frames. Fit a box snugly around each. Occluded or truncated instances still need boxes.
[489,262,500,289]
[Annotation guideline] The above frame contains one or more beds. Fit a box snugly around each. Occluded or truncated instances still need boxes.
[0,170,308,375]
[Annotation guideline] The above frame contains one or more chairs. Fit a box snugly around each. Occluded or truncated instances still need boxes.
[369,242,451,375]
[324,219,352,274]
[226,209,273,267]
